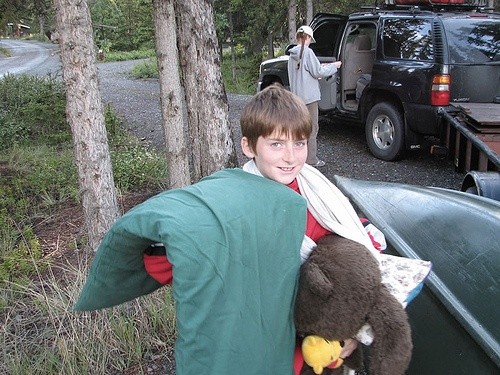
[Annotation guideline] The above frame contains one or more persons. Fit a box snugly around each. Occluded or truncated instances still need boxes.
[288,26,342,166]
[143,83,387,375]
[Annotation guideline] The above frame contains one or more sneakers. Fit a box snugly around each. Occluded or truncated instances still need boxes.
[311,160,326,167]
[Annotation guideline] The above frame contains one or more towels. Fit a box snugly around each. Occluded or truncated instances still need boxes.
[244,159,376,257]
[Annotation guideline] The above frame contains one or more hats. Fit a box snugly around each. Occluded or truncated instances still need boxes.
[296,26,316,44]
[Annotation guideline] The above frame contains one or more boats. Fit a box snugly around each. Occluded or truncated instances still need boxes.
[333,174,500,375]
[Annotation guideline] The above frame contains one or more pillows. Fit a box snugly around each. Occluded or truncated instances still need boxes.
[353,252,433,345]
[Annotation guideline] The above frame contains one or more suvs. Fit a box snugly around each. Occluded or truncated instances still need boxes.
[257,0,500,161]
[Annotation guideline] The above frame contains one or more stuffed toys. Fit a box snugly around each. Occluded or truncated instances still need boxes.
[302,336,345,374]
[296,233,413,375]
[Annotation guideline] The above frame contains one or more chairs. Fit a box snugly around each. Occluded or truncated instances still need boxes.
[355,73,372,97]
[342,35,376,95]
